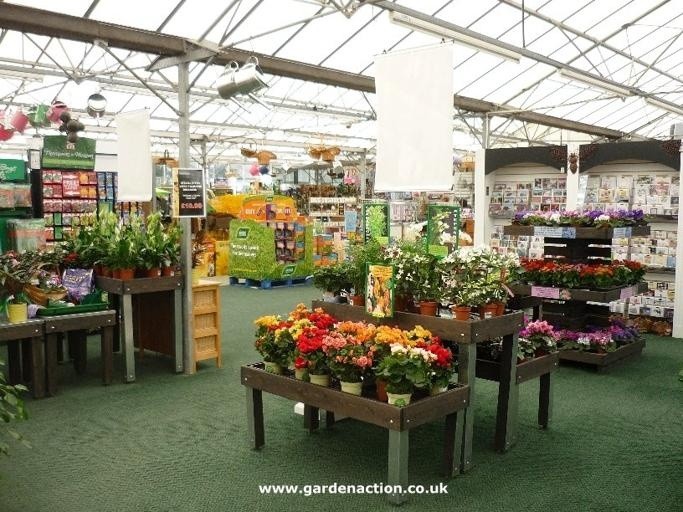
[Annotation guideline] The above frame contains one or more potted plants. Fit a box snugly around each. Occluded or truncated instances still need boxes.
[58,207,188,282]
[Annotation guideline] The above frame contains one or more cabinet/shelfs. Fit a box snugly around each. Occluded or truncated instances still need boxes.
[469,347,560,438]
[95,277,183,385]
[1,313,44,408]
[235,355,474,507]
[45,305,118,404]
[189,277,221,373]
[305,291,526,476]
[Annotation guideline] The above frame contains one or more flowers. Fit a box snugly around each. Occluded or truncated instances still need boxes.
[251,205,649,411]
